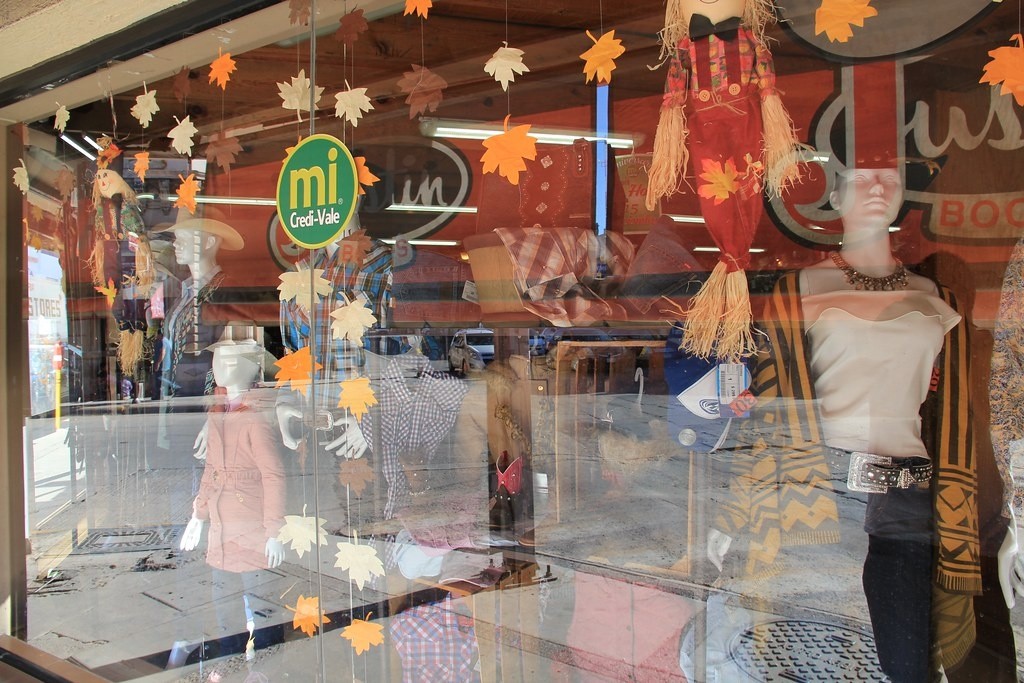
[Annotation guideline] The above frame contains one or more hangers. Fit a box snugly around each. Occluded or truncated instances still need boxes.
[630,367,643,417]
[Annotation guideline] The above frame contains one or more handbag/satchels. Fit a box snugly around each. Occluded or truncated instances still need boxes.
[475,139,628,238]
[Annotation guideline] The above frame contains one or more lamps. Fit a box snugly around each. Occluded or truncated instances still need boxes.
[417,114,646,150]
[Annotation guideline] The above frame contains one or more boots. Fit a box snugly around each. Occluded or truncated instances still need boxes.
[519,457,575,545]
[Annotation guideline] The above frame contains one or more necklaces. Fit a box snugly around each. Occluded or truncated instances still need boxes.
[828,251,909,291]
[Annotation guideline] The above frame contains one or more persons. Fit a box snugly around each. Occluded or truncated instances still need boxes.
[704,146,982,683]
[151,327,174,400]
[645,0,802,360]
[146,203,245,670]
[176,320,288,683]
[86,161,153,376]
[988,237,1024,683]
[275,190,395,683]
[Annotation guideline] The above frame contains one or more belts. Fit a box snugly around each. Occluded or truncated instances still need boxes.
[290,407,352,430]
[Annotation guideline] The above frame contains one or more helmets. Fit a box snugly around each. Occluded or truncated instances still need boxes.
[392,528,443,578]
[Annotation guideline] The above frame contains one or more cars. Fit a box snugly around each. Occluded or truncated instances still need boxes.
[528,326,625,370]
[175,347,214,394]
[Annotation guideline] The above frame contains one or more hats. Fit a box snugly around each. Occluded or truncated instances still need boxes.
[662,321,755,454]
[182,316,281,377]
[807,147,949,225]
[151,204,245,252]
[438,549,504,589]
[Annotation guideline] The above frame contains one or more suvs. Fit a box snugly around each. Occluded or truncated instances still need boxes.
[448,326,497,377]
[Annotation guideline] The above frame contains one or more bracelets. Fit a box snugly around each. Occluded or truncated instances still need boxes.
[408,484,431,496]
